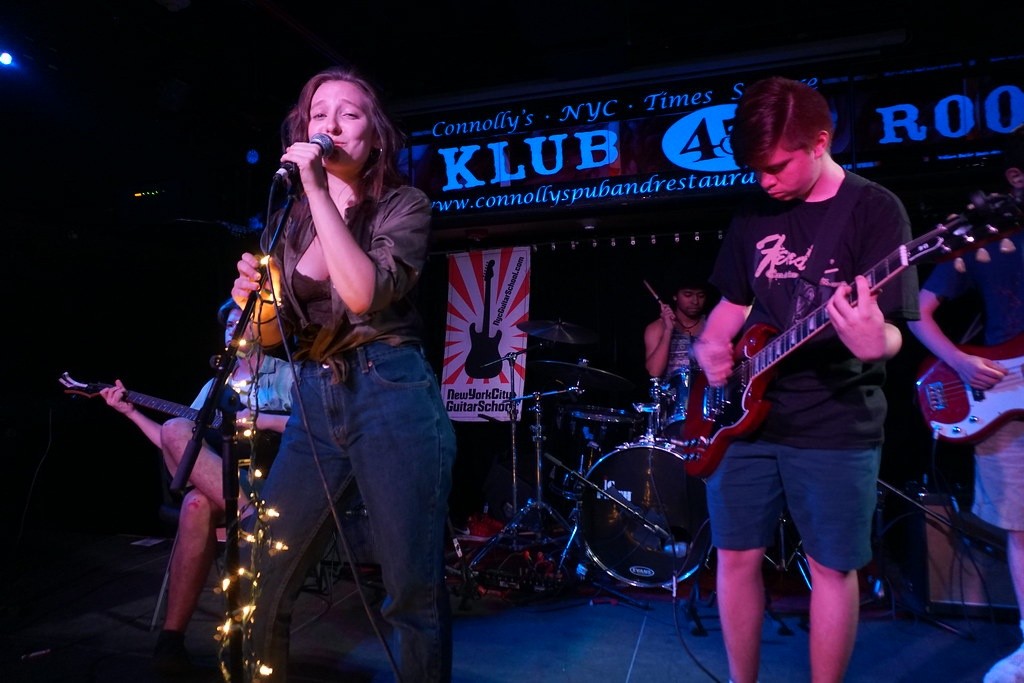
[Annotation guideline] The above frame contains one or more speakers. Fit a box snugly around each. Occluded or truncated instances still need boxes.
[320,482,382,568]
[919,489,1019,615]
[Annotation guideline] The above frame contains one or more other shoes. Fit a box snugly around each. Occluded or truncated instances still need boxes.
[239,511,259,533]
[1016,627,1024,645]
[150,629,190,672]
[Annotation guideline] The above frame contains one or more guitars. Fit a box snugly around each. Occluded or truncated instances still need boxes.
[681,182,1024,479]
[912,336,1023,447]
[53,369,291,460]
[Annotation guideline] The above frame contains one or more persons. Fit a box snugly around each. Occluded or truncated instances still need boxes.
[907,125,1024,682]
[642,272,712,429]
[226,71,453,683]
[97,296,299,683]
[691,78,923,683]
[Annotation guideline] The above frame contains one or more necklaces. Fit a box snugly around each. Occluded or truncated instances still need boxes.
[677,316,700,334]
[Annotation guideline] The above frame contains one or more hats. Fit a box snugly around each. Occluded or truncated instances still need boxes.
[217,297,241,326]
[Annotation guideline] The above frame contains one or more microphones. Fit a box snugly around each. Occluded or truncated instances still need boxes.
[273,133,335,181]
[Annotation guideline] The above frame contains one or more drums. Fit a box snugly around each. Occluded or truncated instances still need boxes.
[567,438,716,593]
[543,400,646,502]
[652,366,690,441]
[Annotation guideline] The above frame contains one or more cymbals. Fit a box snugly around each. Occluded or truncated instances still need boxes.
[514,317,599,346]
[529,358,634,397]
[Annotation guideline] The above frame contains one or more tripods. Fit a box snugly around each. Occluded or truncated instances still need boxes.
[798,477,1009,641]
[468,341,675,608]
